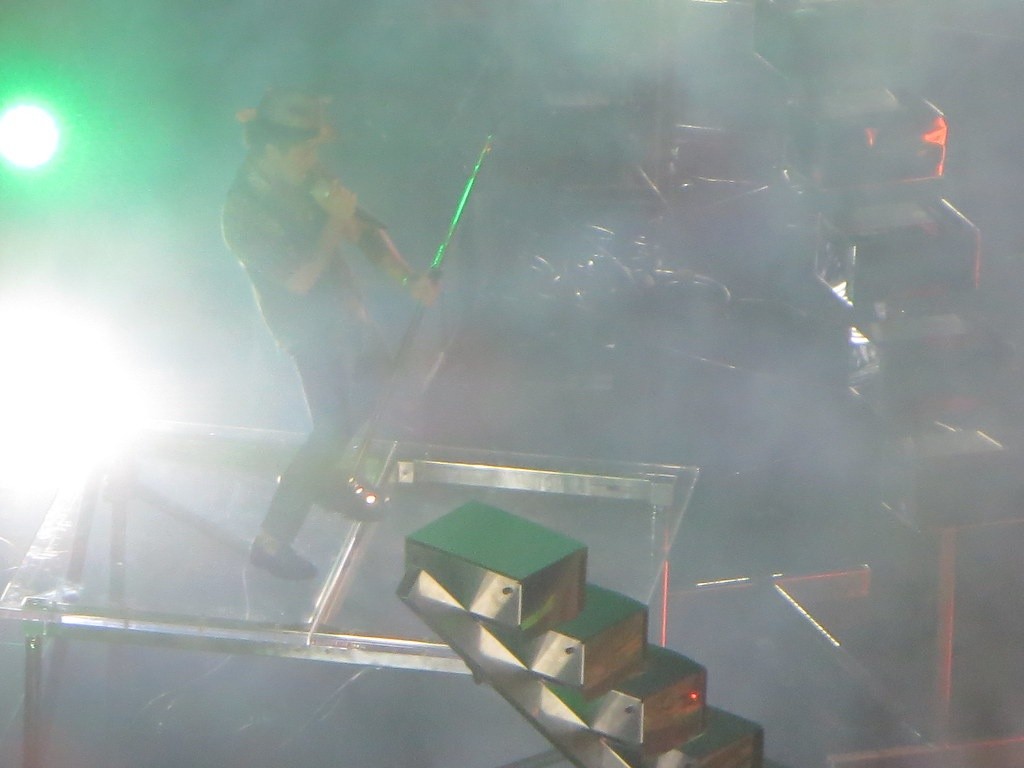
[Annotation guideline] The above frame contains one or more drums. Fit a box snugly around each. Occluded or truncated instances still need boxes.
[656,268,745,379]
[670,113,773,189]
[524,216,657,356]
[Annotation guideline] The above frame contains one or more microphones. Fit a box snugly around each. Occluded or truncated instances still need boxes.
[313,179,390,230]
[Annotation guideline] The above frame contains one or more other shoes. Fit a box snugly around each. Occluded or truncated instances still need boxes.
[322,474,383,515]
[250,537,322,577]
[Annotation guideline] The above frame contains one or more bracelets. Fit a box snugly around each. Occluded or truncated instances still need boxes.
[400,273,420,289]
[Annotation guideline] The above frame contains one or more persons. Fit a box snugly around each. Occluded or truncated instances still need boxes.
[222,86,439,578]
